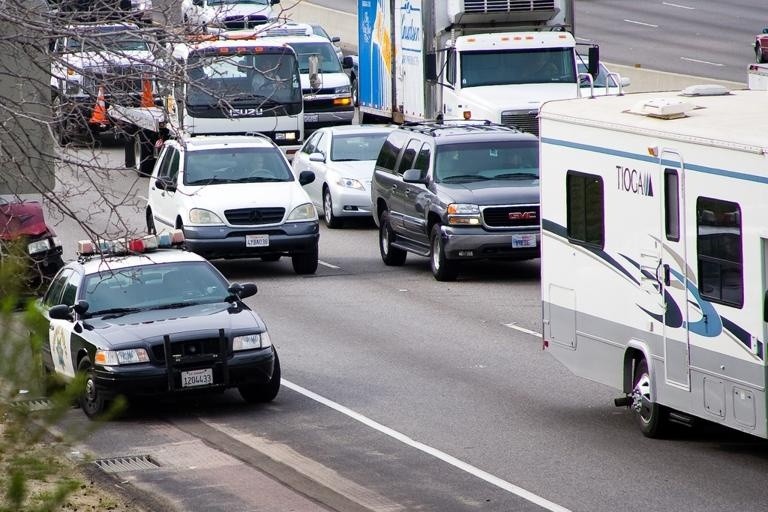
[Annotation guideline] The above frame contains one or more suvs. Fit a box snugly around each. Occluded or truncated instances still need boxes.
[48,21,156,146]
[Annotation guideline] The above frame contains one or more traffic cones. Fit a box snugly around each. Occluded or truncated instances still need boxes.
[89,83,110,125]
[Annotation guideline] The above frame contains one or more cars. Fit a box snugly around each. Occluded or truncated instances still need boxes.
[754,28,768,64]
[577,58,630,95]
[182,0,281,31]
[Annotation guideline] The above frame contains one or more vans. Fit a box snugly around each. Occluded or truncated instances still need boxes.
[252,22,353,123]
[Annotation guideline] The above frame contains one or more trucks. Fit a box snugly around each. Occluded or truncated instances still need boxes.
[108,30,306,177]
[539,84,768,444]
[357,1,581,137]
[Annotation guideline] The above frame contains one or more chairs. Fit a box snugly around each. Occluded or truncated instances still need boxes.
[467,50,557,81]
[84,270,198,313]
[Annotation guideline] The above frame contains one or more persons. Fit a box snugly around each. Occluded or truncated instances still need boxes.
[252,59,286,93]
[521,51,560,82]
